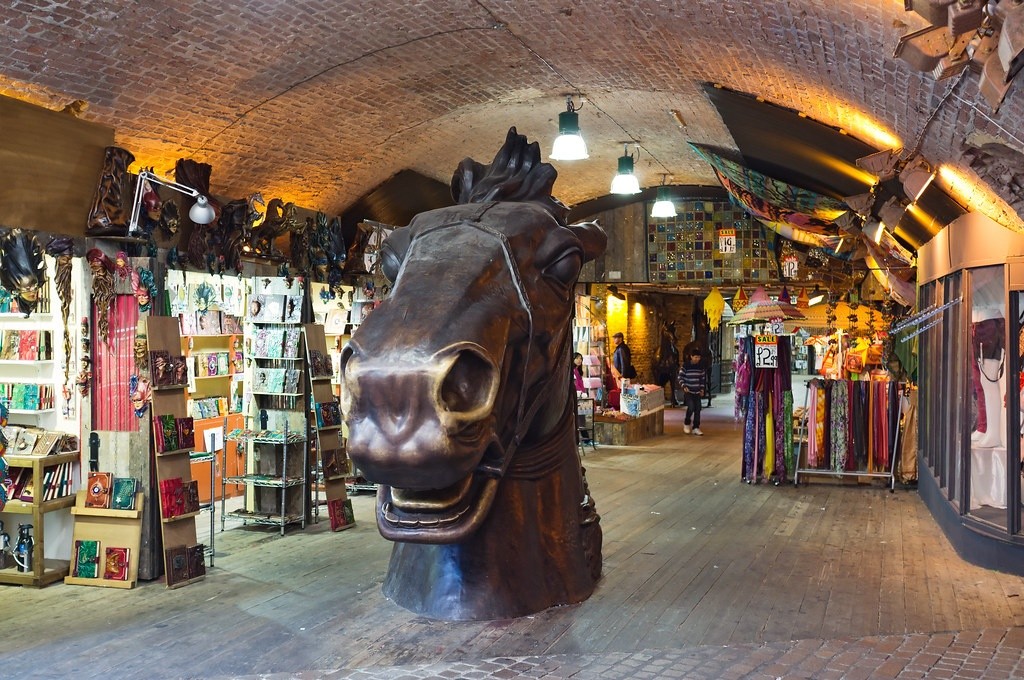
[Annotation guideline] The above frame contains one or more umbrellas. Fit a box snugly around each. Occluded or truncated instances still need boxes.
[726,285,808,327]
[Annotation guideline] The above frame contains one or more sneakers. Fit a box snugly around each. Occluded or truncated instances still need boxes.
[682,421,691,434]
[693,428,704,437]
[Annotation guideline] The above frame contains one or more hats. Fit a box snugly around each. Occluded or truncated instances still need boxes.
[613,333,623,337]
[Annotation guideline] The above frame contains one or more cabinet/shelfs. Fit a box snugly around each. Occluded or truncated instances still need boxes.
[572,318,604,456]
[0,254,389,589]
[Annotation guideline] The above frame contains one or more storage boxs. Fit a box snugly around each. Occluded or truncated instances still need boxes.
[620,388,665,417]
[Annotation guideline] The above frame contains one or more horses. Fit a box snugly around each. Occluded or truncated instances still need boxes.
[334,125,609,624]
[653,321,679,407]
[683,318,713,407]
[248,192,307,255]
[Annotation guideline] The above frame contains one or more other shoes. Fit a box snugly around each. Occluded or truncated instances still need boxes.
[582,441,598,446]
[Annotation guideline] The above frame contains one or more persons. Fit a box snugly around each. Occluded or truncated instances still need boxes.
[135,266,158,312]
[678,349,706,435]
[144,191,179,238]
[573,352,599,446]
[197,282,215,312]
[613,332,631,379]
[311,226,346,275]
[0,228,132,387]
[132,377,152,418]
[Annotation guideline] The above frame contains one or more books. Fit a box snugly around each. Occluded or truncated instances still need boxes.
[71,540,131,581]
[178,293,303,419]
[0,324,72,501]
[310,350,355,528]
[149,349,206,585]
[85,472,137,509]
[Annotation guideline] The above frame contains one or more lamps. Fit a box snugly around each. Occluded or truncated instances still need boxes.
[549,98,589,159]
[834,0,1024,245]
[129,171,215,236]
[807,283,824,307]
[650,175,678,217]
[607,285,626,300]
[610,143,642,194]
